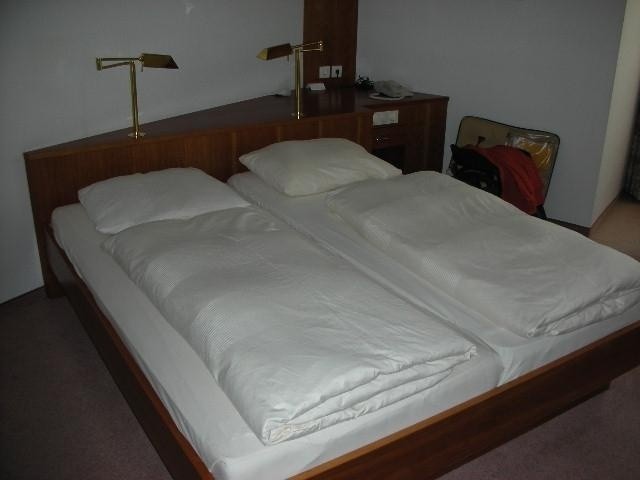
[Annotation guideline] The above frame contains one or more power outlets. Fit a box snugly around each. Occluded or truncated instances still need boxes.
[331,66,342,78]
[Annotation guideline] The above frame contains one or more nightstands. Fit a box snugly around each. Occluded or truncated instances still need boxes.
[359,85,450,177]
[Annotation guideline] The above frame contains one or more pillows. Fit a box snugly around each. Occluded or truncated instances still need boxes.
[237,136,402,198]
[76,166,251,236]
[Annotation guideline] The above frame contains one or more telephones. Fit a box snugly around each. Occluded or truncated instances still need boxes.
[374,79,414,97]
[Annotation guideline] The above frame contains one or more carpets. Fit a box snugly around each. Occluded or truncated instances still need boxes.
[1,284,640,479]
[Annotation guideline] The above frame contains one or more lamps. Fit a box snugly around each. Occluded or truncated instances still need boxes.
[95,53,180,139]
[255,41,324,119]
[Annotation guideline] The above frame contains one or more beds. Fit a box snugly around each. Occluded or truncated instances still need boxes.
[23,93,640,480]
